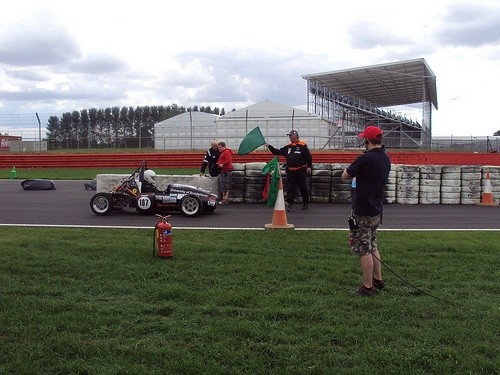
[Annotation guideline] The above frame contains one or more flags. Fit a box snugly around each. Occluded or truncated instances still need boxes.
[238,125,266,155]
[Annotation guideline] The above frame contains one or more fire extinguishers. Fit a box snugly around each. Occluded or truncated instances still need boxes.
[153,214,172,257]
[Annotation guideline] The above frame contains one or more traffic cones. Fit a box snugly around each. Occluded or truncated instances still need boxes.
[477,167,499,206]
[264,177,295,229]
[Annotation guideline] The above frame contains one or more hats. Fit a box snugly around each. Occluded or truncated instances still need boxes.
[287,130,298,135]
[358,126,383,140]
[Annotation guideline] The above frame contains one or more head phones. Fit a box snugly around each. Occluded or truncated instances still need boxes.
[364,138,368,145]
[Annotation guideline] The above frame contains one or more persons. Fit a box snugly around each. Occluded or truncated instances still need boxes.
[265,129,312,212]
[216,142,233,204]
[143,169,165,195]
[200,141,221,202]
[342,126,391,295]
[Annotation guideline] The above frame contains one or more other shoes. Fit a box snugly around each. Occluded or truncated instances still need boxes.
[301,202,308,209]
[219,199,228,205]
[288,203,292,210]
[356,286,374,297]
[373,278,385,289]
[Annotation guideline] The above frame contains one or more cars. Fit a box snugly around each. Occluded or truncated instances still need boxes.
[90,160,219,218]
